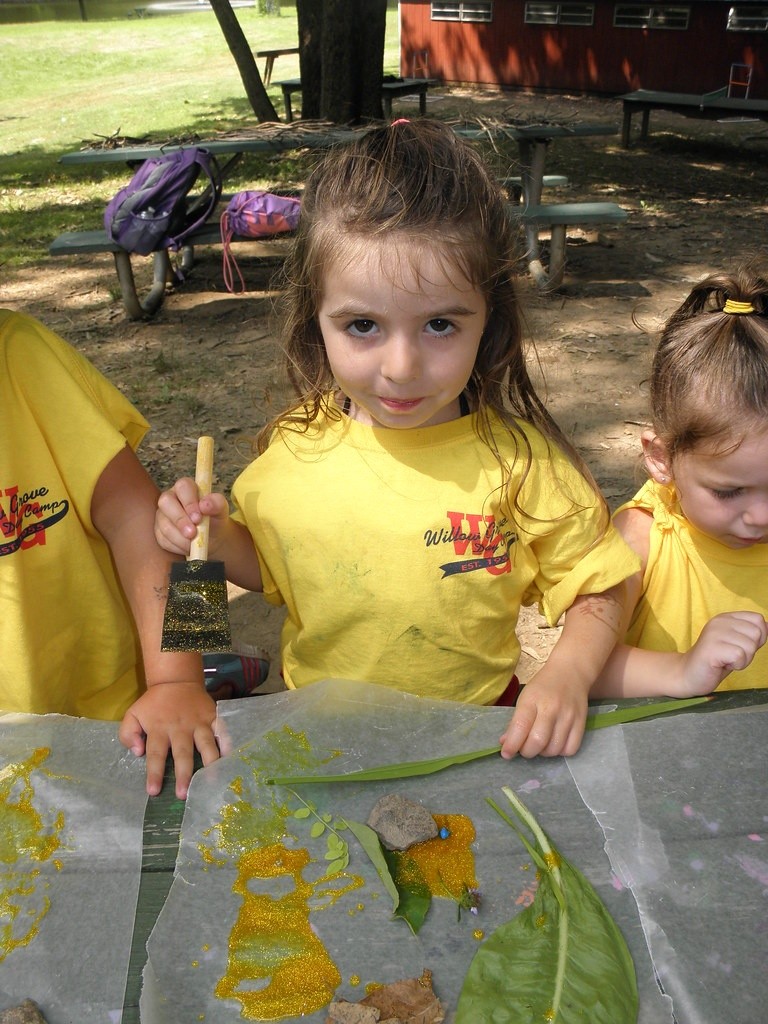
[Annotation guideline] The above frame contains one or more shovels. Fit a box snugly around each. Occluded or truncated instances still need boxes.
[160,435,235,656]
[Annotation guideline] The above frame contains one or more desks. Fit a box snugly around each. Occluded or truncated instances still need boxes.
[61,117,617,277]
[2,688,767,1023]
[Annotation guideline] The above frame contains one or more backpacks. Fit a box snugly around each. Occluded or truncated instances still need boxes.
[101,148,219,255]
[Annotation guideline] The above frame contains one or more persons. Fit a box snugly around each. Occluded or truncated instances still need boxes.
[0,308,244,807]
[149,117,642,763]
[581,267,766,704]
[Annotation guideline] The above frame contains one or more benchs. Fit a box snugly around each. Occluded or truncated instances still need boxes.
[268,75,437,118]
[254,47,299,86]
[49,202,629,320]
[615,89,768,149]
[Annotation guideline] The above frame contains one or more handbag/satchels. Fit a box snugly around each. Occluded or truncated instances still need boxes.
[222,190,303,297]
